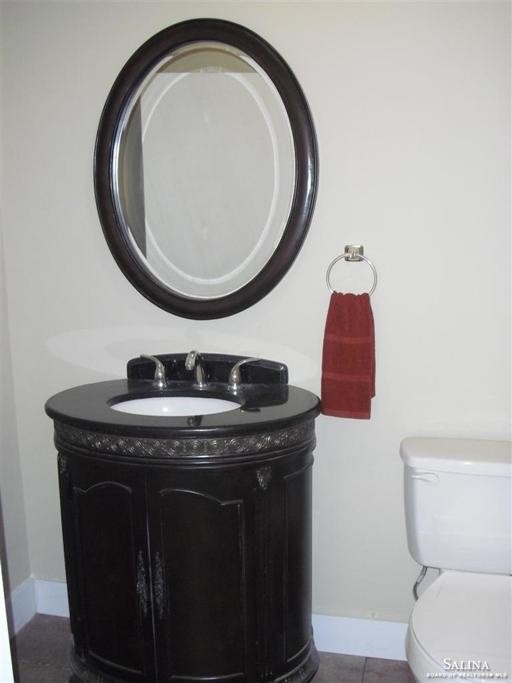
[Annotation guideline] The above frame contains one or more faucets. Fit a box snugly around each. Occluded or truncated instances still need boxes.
[187,415,204,427]
[185,351,208,387]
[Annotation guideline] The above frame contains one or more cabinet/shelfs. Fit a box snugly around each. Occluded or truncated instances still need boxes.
[53,420,318,683]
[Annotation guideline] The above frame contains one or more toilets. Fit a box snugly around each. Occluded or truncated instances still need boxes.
[400,438,512,683]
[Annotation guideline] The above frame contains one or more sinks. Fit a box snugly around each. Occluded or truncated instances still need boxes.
[107,390,245,416]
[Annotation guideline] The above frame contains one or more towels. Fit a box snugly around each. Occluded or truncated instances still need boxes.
[316,290,377,423]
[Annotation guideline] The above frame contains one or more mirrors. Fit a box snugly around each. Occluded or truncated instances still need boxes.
[90,17,321,321]
